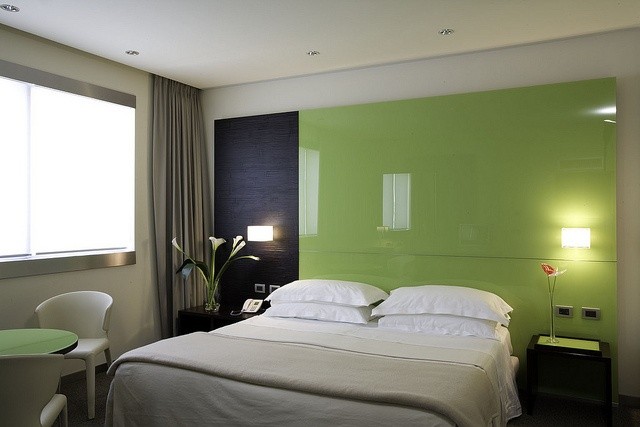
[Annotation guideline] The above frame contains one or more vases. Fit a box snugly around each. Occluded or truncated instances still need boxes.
[544,275,558,344]
[204,282,221,313]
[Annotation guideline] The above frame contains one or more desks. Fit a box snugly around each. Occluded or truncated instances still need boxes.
[0,328,79,356]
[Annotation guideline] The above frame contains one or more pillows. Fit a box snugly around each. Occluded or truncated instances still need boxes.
[263,280,389,307]
[262,308,377,325]
[371,285,514,326]
[377,315,501,338]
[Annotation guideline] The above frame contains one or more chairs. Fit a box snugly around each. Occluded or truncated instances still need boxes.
[33,291,114,420]
[0,354,68,426]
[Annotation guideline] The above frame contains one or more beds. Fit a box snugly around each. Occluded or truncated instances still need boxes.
[104,316,522,427]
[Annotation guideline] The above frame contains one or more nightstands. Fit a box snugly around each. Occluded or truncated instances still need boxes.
[525,335,613,427]
[177,301,265,335]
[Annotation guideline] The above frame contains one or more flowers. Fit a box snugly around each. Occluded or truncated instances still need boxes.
[162,235,261,281]
[539,262,557,341]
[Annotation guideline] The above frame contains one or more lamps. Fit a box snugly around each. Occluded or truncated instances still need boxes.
[560,227,591,249]
[247,225,273,243]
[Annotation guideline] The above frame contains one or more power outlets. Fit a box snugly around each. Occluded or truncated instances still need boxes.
[270,285,280,293]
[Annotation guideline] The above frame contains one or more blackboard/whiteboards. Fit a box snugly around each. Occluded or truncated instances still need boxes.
[241,298,263,313]
